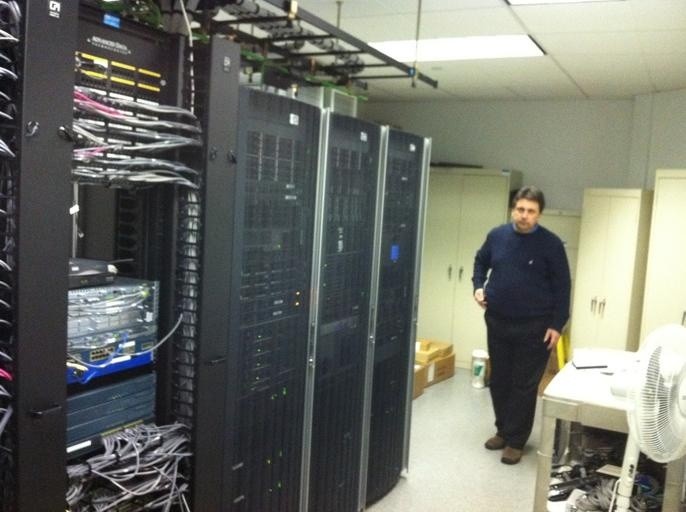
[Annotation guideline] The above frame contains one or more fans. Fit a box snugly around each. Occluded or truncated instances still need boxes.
[607,324,684,510]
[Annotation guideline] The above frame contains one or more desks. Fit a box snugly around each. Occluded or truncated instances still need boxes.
[531,349,685,512]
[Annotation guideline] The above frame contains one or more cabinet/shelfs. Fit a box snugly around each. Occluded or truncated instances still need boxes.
[571,188,652,357]
[637,166,686,347]
[537,206,581,329]
[417,167,522,369]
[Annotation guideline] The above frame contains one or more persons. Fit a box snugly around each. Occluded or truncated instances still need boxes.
[473,185,571,464]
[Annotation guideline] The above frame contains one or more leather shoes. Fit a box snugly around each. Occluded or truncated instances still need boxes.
[485,436,521,465]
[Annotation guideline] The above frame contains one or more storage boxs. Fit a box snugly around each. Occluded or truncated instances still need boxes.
[413,340,456,402]
